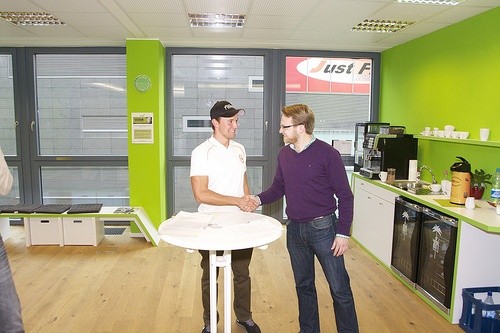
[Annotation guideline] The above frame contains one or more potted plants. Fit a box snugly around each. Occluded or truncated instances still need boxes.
[469,169,493,200]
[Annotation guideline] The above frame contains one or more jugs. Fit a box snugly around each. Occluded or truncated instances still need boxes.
[449,155,472,205]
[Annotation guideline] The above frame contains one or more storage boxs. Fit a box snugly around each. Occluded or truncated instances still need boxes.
[458,286,500,333]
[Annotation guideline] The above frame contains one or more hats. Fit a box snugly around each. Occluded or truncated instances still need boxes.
[210,101,245,120]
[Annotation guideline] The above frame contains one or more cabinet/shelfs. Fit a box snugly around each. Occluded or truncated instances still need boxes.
[349,175,399,270]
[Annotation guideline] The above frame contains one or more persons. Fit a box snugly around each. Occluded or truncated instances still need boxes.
[191,100,262,333]
[0,144,25,333]
[243,104,360,333]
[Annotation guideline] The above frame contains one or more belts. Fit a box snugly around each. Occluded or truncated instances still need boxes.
[312,213,334,220]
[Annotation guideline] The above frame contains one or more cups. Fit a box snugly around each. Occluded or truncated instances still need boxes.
[378,172,388,183]
[464,196,475,209]
[427,180,452,195]
[387,168,396,182]
[479,128,490,141]
[419,125,469,139]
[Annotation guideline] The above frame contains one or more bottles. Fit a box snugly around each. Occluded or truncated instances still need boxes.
[481,291,495,319]
[489,168,500,204]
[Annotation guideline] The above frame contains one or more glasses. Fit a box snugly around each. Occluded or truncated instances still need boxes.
[281,123,305,130]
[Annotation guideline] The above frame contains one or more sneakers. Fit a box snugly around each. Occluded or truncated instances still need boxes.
[202,325,210,333]
[236,318,261,333]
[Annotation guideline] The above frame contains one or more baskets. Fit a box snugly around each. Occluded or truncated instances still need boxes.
[459,287,500,333]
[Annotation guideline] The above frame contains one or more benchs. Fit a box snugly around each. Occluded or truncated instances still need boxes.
[0,206,158,248]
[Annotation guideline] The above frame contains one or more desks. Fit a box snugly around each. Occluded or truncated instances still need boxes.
[158,212,283,333]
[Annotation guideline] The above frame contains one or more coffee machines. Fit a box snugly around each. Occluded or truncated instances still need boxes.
[359,133,418,179]
